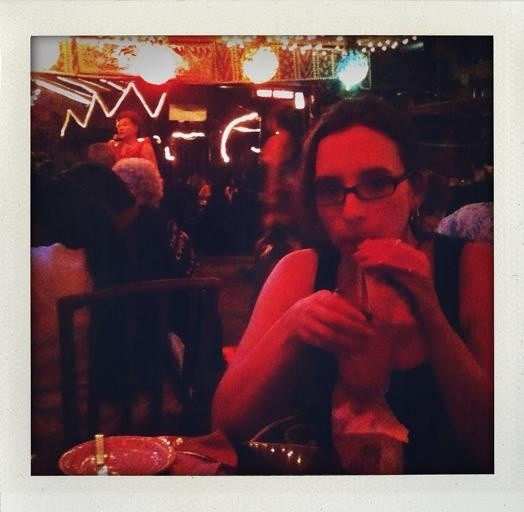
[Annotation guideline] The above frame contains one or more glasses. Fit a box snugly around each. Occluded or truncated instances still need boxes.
[244,409,328,475]
[311,162,422,207]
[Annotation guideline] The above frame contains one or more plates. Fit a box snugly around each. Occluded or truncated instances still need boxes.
[57,434,178,475]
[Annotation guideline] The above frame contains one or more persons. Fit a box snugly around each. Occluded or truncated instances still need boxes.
[30,95,494,453]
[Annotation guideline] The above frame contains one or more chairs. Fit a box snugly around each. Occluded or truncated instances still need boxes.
[56,276,223,438]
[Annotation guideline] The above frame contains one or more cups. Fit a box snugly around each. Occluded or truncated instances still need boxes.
[333,288,393,402]
